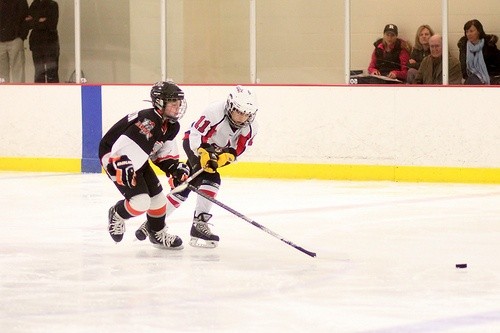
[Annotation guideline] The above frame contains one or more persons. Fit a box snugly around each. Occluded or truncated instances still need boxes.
[99,80,190,249]
[368,19,499,87]
[1,1,61,84]
[135,84,259,242]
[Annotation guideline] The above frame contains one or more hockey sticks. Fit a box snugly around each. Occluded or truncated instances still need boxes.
[178,178,317,257]
[169,165,207,195]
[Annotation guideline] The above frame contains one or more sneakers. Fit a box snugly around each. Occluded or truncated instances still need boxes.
[107,205,130,246]
[134,220,149,240]
[145,222,184,250]
[189,211,219,248]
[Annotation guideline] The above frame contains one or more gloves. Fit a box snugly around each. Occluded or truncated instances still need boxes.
[196,143,218,173]
[112,155,136,189]
[168,163,190,188]
[218,148,236,168]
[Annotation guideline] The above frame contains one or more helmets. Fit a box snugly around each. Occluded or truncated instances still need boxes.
[224,86,260,129]
[150,81,187,123]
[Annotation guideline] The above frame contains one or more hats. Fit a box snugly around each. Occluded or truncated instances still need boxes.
[384,24,398,34]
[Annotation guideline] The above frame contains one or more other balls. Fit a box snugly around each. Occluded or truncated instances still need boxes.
[456,264,467,269]
